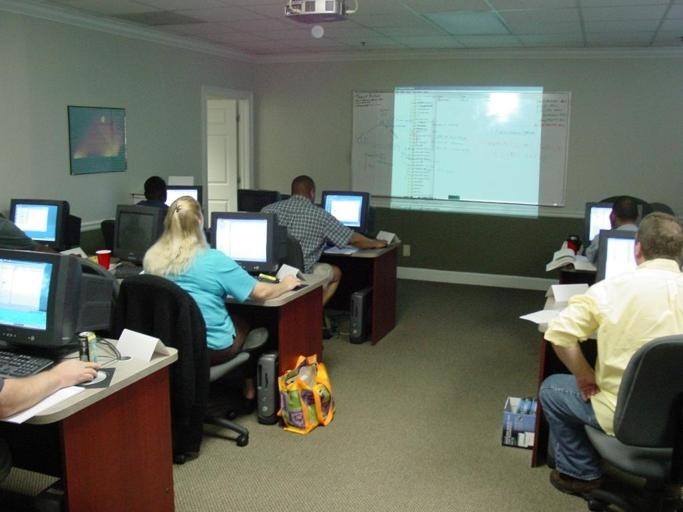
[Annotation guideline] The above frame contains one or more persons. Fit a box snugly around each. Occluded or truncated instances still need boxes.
[0,359,102,485]
[142,195,302,400]
[0,212,61,252]
[587,199,646,261]
[539,210,683,496]
[135,175,171,240]
[256,176,388,331]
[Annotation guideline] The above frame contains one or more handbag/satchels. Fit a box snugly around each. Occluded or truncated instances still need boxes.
[278,355,337,435]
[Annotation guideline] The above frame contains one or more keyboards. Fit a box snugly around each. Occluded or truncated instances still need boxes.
[0,349,55,381]
[113,265,144,279]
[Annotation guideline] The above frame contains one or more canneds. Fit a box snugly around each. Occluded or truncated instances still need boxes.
[77,331,97,362]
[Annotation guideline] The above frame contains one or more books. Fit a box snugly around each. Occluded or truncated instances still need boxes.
[544,247,598,271]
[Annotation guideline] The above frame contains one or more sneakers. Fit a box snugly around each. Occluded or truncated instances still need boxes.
[550,469,600,500]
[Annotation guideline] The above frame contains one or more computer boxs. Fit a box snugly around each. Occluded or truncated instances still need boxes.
[350,286,372,344]
[257,349,279,424]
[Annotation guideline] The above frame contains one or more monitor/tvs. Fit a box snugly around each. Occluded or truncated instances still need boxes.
[585,202,643,249]
[594,229,640,285]
[210,210,288,275]
[237,189,291,213]
[0,249,113,356]
[9,184,203,263]
[321,190,370,235]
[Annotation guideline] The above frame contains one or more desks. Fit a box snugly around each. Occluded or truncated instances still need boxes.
[531,271,596,467]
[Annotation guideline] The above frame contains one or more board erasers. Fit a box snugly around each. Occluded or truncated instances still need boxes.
[449,196,460,200]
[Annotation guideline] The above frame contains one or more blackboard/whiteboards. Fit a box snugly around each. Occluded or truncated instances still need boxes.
[350,88,571,208]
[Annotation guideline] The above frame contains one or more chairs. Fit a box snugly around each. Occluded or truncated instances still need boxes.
[584,334,683,512]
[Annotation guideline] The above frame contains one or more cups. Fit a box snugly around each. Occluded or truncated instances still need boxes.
[95,249,111,271]
[566,235,580,255]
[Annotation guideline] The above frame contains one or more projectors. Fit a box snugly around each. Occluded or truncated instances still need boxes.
[284,0,351,24]
